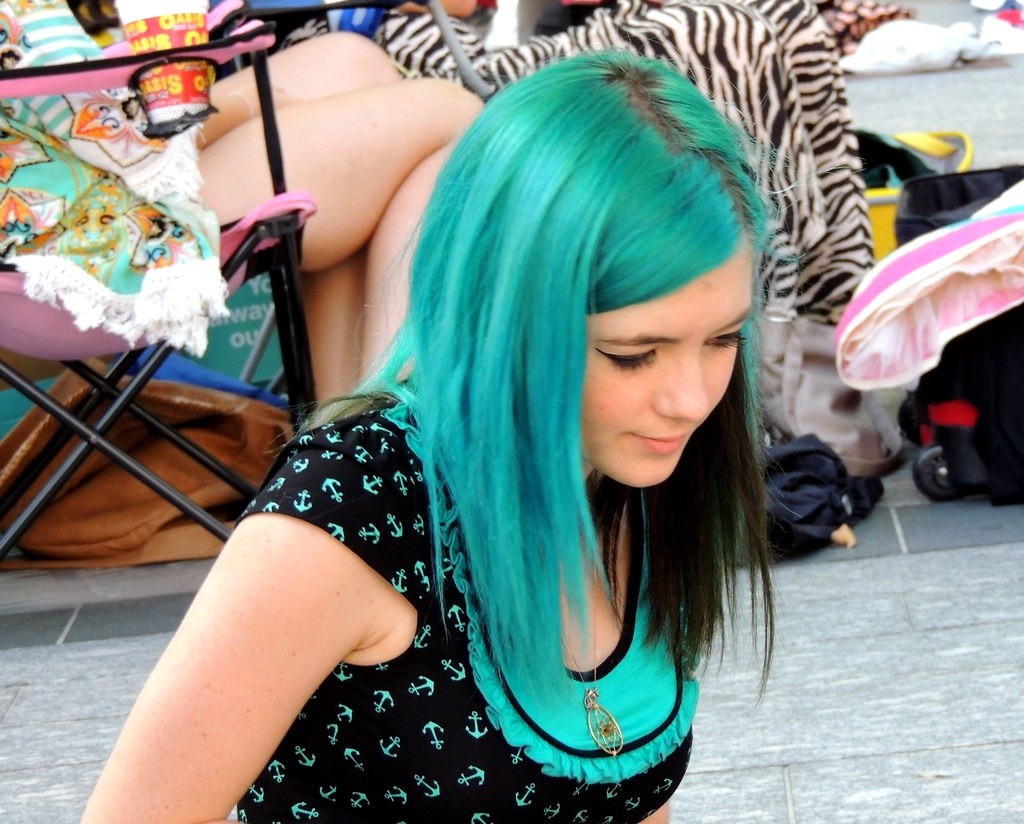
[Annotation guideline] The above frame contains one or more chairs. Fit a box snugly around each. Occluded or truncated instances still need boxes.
[0,0,318,576]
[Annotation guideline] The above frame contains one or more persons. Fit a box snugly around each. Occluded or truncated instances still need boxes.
[374,0,878,437]
[80,51,775,824]
[2,0,486,408]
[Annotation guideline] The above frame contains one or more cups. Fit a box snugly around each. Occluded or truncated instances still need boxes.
[114,0,210,126]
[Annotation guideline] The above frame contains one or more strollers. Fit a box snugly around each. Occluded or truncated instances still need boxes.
[891,164,1024,506]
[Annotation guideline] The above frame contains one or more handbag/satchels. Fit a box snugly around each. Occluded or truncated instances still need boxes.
[1,355,301,571]
[758,417,885,560]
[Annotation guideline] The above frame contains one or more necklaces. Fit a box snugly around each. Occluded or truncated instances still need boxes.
[562,564,623,755]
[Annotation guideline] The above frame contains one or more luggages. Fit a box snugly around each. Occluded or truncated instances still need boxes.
[896,164,1023,506]
